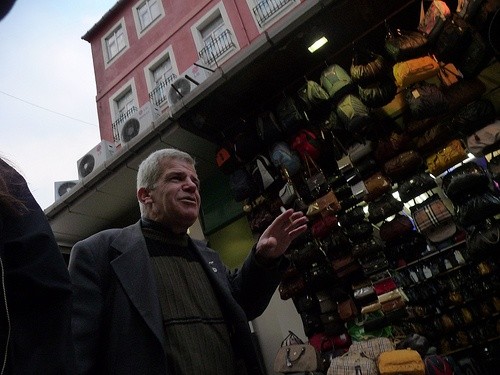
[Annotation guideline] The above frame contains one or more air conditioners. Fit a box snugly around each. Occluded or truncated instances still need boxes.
[118,101,162,147]
[77,140,117,181]
[162,59,214,108]
[54,181,78,201]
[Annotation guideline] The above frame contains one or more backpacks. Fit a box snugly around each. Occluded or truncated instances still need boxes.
[269,142,302,180]
[230,161,262,203]
[249,154,281,192]
[333,94,369,131]
[406,80,450,121]
[320,63,353,100]
[297,78,330,113]
[288,130,324,169]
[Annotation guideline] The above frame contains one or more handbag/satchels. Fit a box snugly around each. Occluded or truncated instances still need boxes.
[393,55,440,91]
[379,212,414,245]
[383,19,430,62]
[274,333,318,372]
[368,192,403,224]
[453,190,500,226]
[441,162,490,205]
[417,0,451,40]
[425,138,467,177]
[398,171,438,203]
[348,336,395,361]
[279,180,296,206]
[376,349,425,375]
[410,190,454,236]
[350,42,384,81]
[327,350,378,374]
[213,1,500,375]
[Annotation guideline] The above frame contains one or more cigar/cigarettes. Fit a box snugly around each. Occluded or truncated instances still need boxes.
[280,206,293,222]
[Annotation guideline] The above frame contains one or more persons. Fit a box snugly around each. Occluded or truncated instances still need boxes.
[67,148,308,375]
